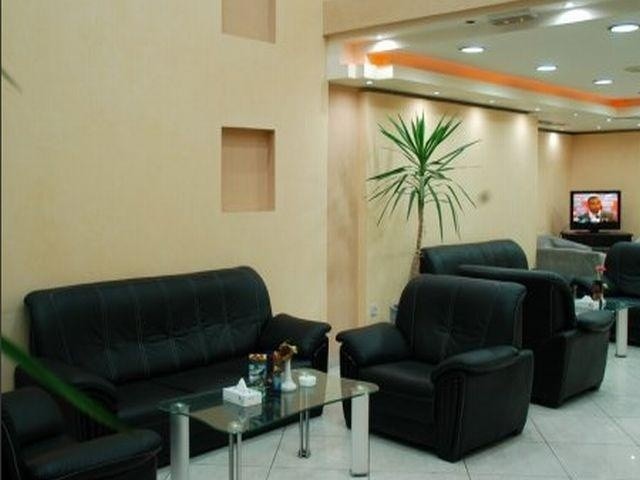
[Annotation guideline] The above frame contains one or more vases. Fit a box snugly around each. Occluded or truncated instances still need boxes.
[280,358,296,392]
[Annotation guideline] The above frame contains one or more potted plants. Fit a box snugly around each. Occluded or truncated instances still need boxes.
[362,106,487,325]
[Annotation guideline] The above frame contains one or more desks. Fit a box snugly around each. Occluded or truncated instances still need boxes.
[560,232,634,248]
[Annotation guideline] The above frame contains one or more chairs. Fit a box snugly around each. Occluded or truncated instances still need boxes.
[456,264,614,409]
[570,240,640,346]
[1,386,162,480]
[336,274,533,463]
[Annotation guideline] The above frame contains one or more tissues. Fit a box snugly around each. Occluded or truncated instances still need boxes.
[223,378,263,407]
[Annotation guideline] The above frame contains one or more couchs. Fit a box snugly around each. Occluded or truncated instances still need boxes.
[16,265,331,469]
[417,240,527,276]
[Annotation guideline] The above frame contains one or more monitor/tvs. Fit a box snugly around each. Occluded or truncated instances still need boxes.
[570,191,621,234]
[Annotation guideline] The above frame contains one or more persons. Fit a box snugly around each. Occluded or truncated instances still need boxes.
[577,196,614,223]
